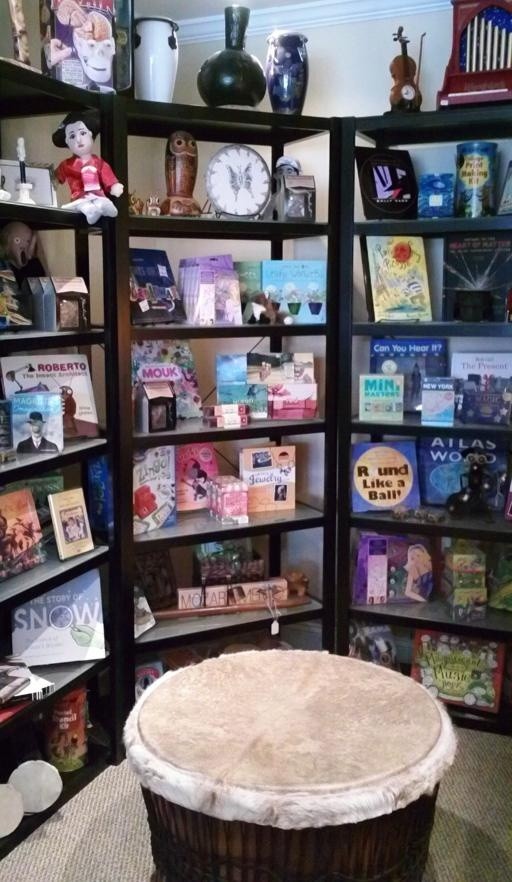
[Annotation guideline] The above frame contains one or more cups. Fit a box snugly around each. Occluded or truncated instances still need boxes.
[134,16,180,104]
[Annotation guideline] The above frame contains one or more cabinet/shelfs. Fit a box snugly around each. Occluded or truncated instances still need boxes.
[0,59,511,859]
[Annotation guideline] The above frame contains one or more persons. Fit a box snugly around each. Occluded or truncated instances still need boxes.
[47,102,126,225]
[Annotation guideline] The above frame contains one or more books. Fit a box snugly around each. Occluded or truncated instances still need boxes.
[0,351,101,712]
[348,235,511,718]
[127,247,328,708]
[40,0,116,94]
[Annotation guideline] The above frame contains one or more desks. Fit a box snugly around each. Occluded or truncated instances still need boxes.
[122,650,458,882]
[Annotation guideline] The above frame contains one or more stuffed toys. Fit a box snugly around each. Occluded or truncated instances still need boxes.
[1,221,50,311]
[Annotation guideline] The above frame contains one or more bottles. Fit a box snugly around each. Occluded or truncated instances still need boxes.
[43,682,92,774]
[454,140,498,220]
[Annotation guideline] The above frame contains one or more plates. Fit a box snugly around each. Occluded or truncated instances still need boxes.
[204,142,274,222]
